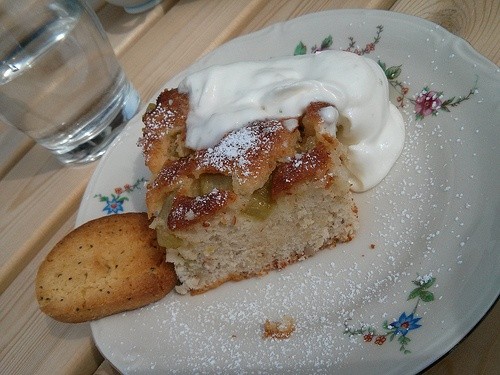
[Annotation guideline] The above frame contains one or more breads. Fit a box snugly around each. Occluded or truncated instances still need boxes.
[137,49,406,297]
[35,212,178,323]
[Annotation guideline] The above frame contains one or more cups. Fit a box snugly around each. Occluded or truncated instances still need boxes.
[1,0,141,166]
[75,8,500,374]
